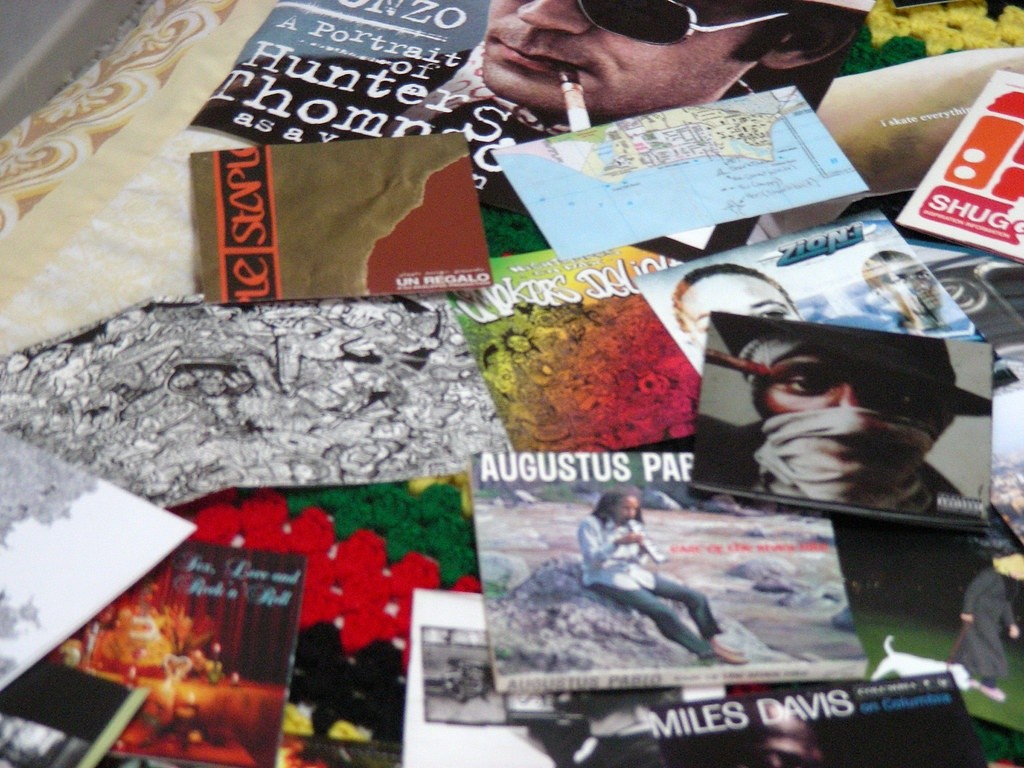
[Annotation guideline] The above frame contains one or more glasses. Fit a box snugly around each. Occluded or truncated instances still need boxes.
[578,1,790,46]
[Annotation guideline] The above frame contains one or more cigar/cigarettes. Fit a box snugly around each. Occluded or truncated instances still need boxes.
[554,62,592,135]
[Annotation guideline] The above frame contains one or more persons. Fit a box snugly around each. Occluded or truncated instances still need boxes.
[578,486,749,668]
[194,0,865,266]
[953,553,1024,703]
[657,696,824,768]
[692,311,993,523]
[668,266,808,354]
[862,251,954,333]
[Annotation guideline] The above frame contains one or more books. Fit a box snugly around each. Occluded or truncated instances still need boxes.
[0,0,1024,768]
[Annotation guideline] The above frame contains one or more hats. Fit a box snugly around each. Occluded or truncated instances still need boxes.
[713,312,993,417]
